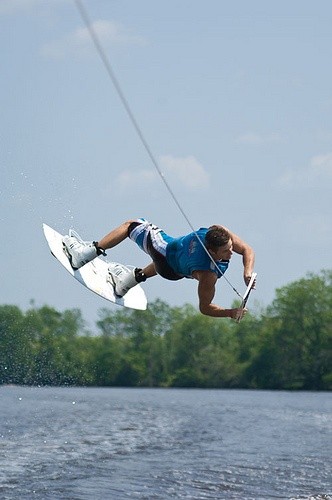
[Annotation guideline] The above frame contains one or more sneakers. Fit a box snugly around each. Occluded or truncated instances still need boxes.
[64,235,101,271]
[107,263,139,297]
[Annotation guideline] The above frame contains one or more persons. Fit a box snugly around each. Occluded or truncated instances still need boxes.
[63,218,258,319]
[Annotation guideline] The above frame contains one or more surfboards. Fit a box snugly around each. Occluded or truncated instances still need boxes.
[42,222,149,311]
